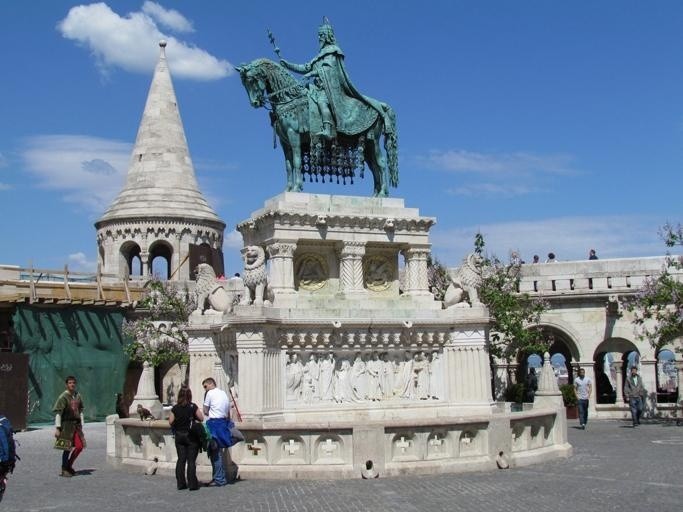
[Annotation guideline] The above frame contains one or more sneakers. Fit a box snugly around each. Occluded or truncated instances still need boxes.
[204,480,218,486]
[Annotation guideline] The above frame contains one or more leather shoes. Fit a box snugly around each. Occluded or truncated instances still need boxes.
[61,470,72,477]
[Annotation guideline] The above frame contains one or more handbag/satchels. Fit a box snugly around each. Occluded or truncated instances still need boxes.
[187,404,207,453]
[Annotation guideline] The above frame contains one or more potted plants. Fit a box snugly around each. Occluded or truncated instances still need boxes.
[558,383,582,419]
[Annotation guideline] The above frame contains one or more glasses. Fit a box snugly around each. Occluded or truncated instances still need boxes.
[203,386,206,388]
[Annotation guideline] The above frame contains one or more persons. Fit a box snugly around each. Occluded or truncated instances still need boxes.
[510,249,599,265]
[624,367,644,427]
[235,273,240,277]
[526,368,538,402]
[573,368,592,430]
[281,24,391,141]
[52,376,87,477]
[286,351,439,401]
[202,378,230,486]
[217,273,225,280]
[168,387,204,490]
[0,415,20,502]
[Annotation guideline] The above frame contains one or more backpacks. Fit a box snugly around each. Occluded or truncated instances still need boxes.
[0,414,20,482]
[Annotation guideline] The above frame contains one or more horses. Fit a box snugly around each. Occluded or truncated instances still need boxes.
[234,57,399,197]
